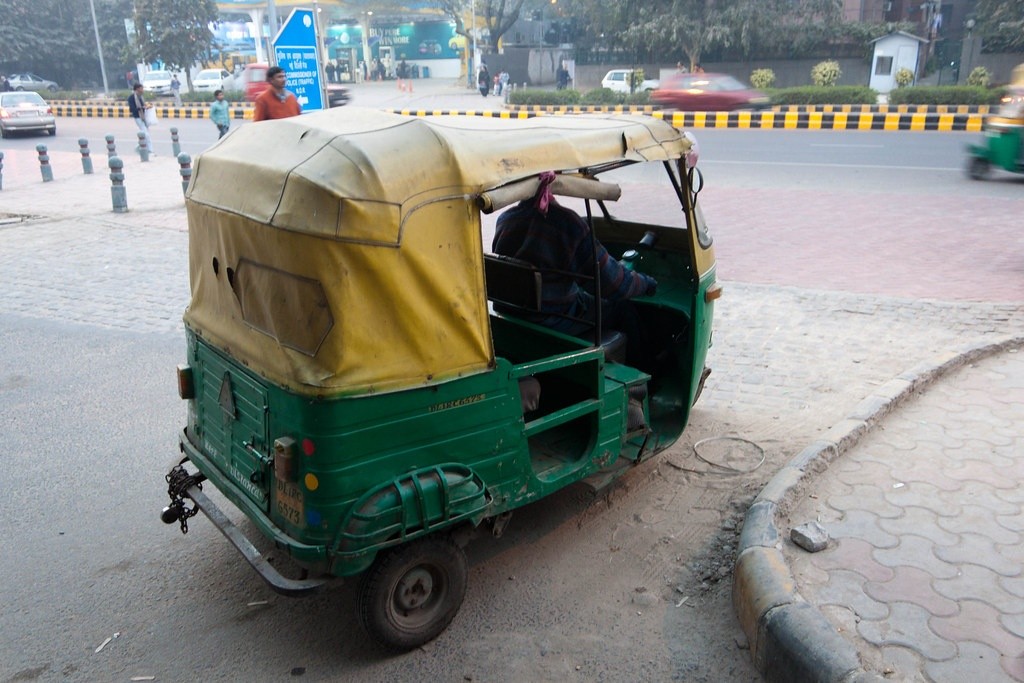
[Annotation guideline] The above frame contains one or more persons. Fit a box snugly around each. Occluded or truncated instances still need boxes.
[1008,52,1024,96]
[677,60,704,73]
[170,74,181,108]
[555,64,569,90]
[1,75,10,91]
[325,55,408,83]
[478,60,510,96]
[128,84,153,154]
[209,90,230,140]
[492,197,658,335]
[253,67,300,121]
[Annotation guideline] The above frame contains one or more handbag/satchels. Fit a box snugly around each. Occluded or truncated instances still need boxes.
[144,106,161,127]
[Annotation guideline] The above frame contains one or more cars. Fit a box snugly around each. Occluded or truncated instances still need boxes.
[4,73,59,92]
[0,92,56,138]
[191,69,234,97]
[233,64,347,109]
[659,74,770,107]
[600,70,659,93]
[140,71,176,96]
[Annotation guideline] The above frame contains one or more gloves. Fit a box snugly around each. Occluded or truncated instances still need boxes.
[644,275,659,297]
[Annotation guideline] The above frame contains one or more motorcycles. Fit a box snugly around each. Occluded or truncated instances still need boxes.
[964,83,1024,178]
[169,109,721,650]
